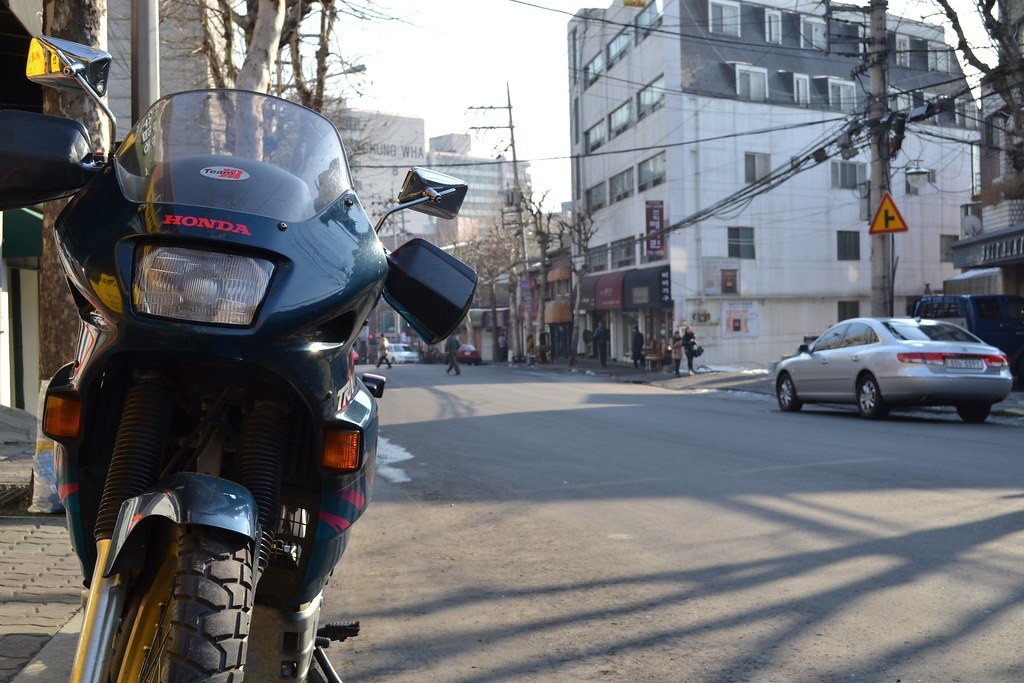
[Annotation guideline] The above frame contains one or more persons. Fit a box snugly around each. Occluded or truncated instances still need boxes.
[375,333,393,368]
[682,326,698,375]
[631,326,646,368]
[417,340,426,360]
[498,333,507,362]
[672,331,683,377]
[593,322,610,368]
[445,331,461,376]
[376,340,392,370]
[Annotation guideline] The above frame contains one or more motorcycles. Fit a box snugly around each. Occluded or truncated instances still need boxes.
[0,34,482,683]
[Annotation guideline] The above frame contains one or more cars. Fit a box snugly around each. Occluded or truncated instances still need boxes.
[456,343,482,366]
[775,316,1014,423]
[378,343,420,364]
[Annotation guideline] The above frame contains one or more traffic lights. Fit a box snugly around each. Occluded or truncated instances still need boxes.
[368,332,375,344]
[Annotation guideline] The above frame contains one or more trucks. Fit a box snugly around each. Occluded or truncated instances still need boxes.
[803,294,1023,392]
